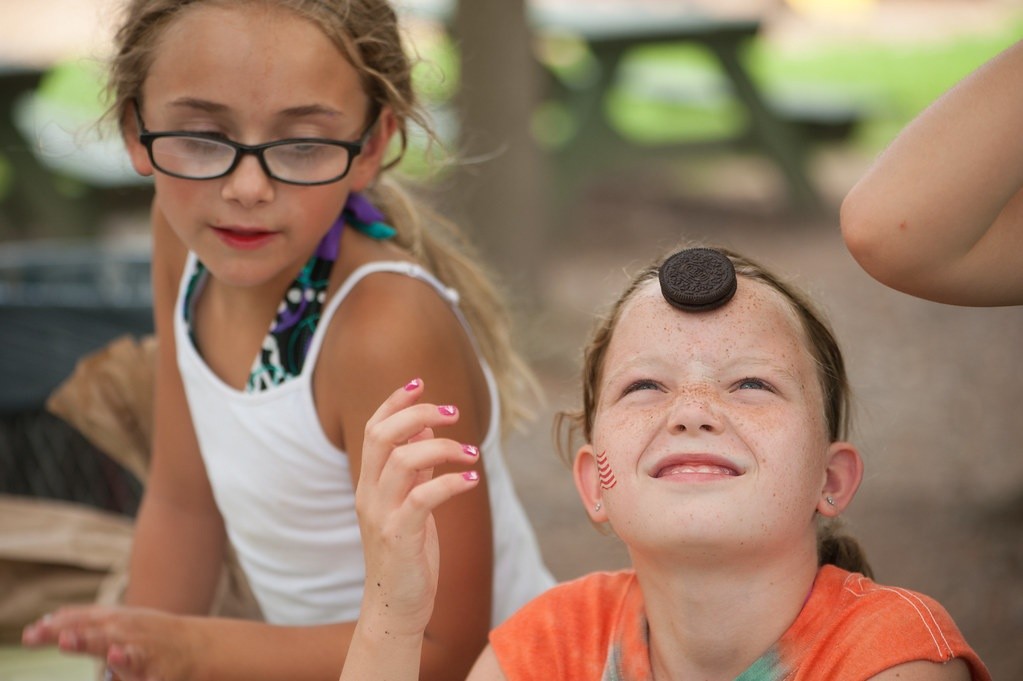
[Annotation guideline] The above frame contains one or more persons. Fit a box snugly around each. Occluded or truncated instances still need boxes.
[840,39,1022,307]
[23,3,561,681]
[338,248,997,681]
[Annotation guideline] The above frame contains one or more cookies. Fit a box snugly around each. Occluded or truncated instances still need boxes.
[658,247,737,311]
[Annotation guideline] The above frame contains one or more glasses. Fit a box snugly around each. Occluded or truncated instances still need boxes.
[131,97,380,186]
